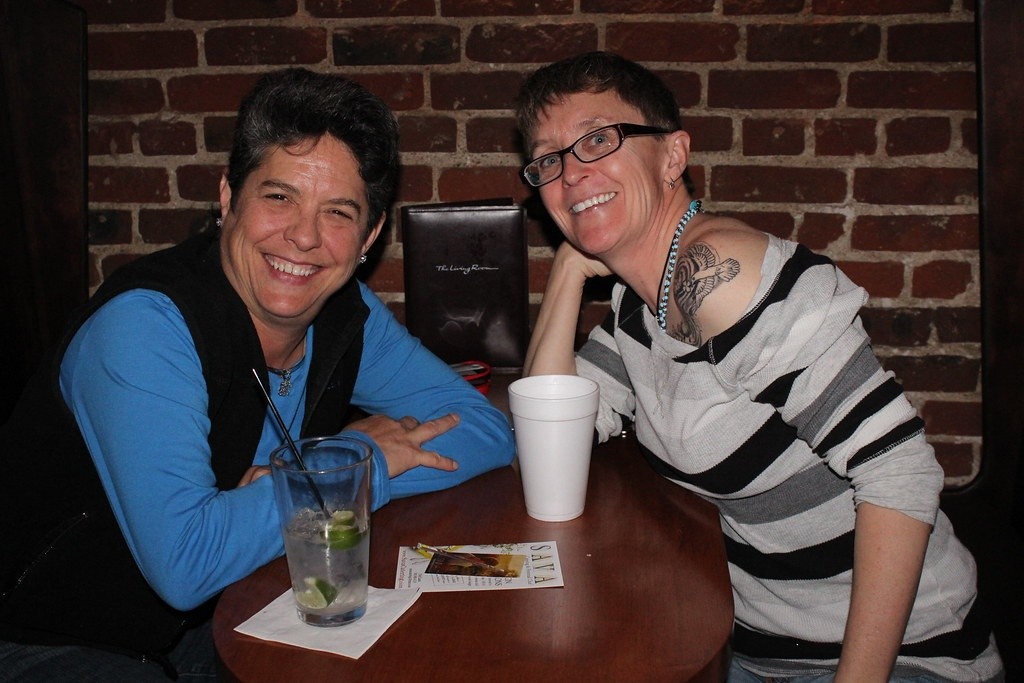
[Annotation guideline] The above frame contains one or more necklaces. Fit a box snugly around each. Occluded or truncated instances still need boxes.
[656,200,704,335]
[267,355,305,397]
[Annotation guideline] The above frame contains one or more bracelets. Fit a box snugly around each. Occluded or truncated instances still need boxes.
[268,456,288,467]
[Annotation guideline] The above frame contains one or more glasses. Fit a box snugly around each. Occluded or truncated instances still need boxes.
[523,123,672,188]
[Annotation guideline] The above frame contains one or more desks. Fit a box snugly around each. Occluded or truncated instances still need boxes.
[212,466,733,683]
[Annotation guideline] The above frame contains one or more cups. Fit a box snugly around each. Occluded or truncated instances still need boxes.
[507,374,600,522]
[269,435,374,629]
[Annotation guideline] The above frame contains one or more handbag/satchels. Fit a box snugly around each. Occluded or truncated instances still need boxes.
[401,197,534,378]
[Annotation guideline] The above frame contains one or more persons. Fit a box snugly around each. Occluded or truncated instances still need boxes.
[437,552,516,577]
[0,67,517,683]
[511,52,1004,683]
